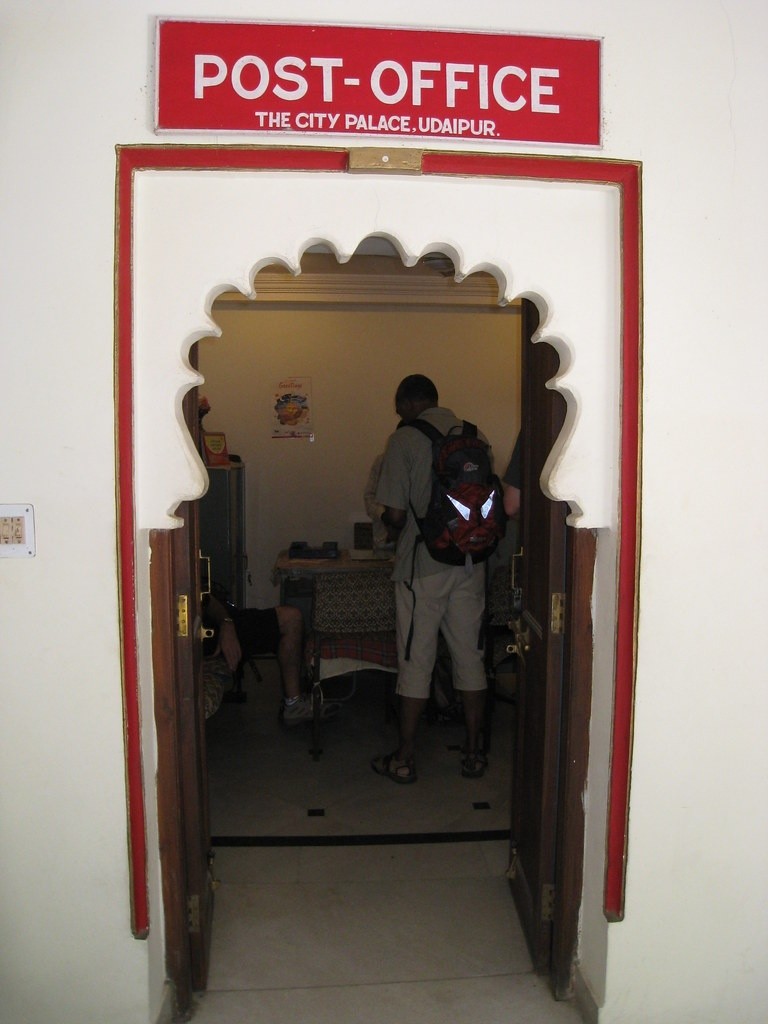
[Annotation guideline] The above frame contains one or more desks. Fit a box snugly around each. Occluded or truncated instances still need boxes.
[272,550,392,719]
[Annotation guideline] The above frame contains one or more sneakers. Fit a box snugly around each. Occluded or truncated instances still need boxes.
[283,696,342,722]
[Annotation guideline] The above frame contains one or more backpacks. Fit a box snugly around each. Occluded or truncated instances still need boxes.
[393,417,509,567]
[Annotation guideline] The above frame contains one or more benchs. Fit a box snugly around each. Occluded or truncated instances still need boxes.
[204,655,246,719]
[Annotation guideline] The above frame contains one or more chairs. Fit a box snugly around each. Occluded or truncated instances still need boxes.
[299,567,396,763]
[427,581,520,752]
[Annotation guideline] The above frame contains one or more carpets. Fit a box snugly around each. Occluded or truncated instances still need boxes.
[205,878,536,992]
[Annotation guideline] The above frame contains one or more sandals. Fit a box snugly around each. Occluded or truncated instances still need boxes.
[462,753,488,777]
[370,752,416,784]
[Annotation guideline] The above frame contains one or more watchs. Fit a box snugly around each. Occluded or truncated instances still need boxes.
[220,616,234,625]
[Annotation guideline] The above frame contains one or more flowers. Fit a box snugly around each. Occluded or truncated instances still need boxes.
[198,395,211,411]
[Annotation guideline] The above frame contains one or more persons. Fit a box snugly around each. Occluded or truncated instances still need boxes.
[369,374,522,784]
[199,577,344,732]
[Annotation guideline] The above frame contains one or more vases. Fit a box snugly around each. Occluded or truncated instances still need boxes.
[199,409,206,457]
